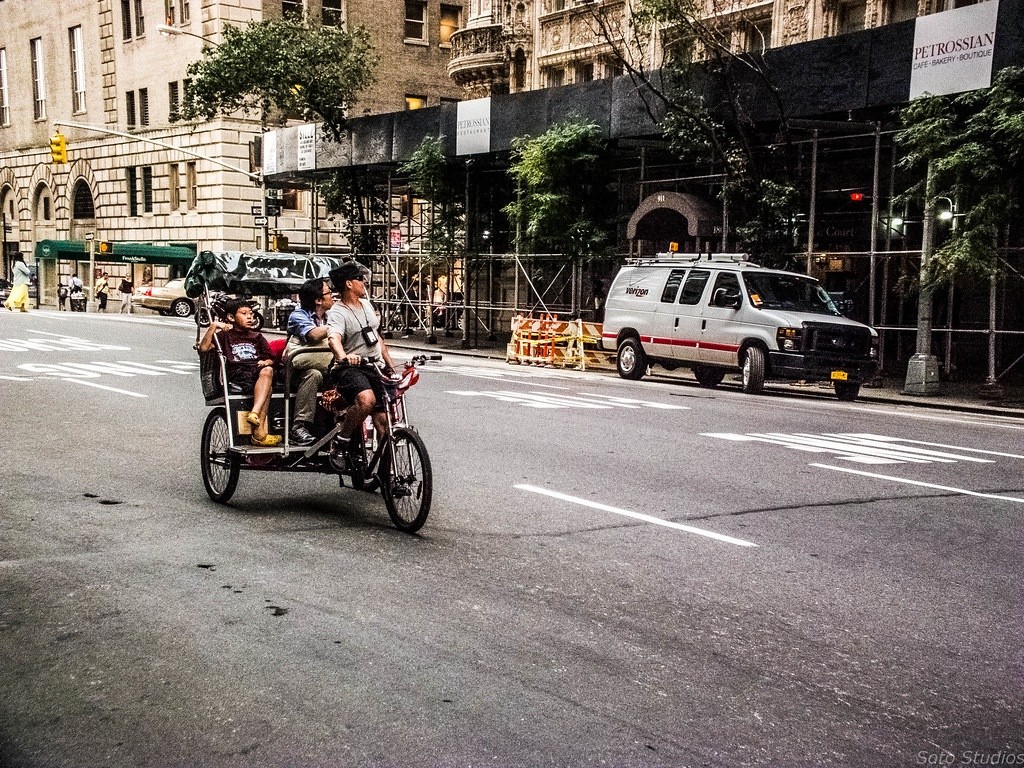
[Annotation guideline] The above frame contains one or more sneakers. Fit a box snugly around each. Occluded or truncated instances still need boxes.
[391,475,406,493]
[251,434,282,446]
[246,412,260,427]
[329,434,351,470]
[289,425,318,445]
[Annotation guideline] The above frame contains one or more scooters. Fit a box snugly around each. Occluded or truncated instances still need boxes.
[194,289,265,332]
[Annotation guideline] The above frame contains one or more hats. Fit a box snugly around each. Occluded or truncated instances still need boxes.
[328,260,369,290]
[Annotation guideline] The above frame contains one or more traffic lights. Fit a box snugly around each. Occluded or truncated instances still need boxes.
[99,241,114,255]
[49,134,68,165]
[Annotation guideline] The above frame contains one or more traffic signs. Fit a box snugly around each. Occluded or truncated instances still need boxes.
[251,204,282,217]
[253,216,269,227]
[85,233,94,240]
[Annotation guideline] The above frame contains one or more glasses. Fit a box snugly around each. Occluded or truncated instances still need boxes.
[323,289,333,296]
[352,276,363,281]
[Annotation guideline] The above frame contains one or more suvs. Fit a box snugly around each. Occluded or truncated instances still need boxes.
[601,250,883,402]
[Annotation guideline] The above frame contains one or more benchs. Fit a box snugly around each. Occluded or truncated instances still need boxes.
[193,339,323,407]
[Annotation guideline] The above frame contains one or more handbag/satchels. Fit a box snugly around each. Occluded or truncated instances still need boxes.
[96,292,100,299]
[286,335,334,370]
[118,284,124,291]
[71,285,82,293]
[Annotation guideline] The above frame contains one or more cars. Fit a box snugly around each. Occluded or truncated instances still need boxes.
[27,285,38,298]
[0,278,13,307]
[267,290,465,332]
[133,277,237,317]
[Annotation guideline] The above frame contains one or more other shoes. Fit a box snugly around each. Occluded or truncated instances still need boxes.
[3,303,12,312]
[20,309,27,312]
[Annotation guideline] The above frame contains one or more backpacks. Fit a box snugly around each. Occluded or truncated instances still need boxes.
[60,288,67,297]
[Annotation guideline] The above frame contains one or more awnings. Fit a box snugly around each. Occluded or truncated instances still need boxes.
[34,239,196,312]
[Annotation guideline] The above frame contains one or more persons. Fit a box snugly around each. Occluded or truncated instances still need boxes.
[3,252,32,312]
[200,299,282,447]
[57,283,68,311]
[95,272,112,313]
[119,273,135,316]
[327,262,400,460]
[68,273,83,311]
[281,277,341,449]
[143,281,152,296]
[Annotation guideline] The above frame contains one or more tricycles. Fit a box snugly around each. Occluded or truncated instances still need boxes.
[183,248,443,535]
